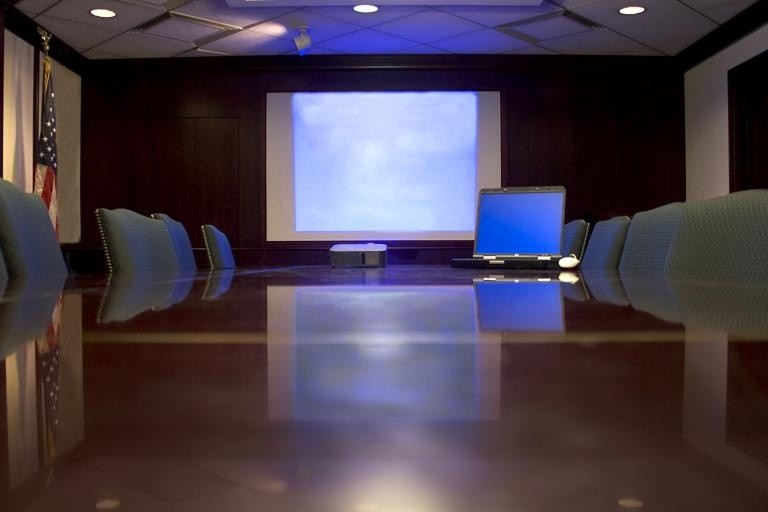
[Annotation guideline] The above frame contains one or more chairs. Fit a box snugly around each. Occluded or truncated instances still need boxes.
[201,266,235,301]
[199,224,238,271]
[148,269,196,312]
[150,211,197,274]
[0,276,69,362]
[579,266,632,308]
[92,271,180,325]
[665,278,768,343]
[561,219,591,262]
[617,271,682,326]
[616,202,686,275]
[0,176,70,278]
[579,215,632,269]
[663,188,768,288]
[94,207,180,273]
[554,267,592,304]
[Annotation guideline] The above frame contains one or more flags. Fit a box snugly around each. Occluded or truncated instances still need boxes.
[32,52,60,247]
[35,293,62,476]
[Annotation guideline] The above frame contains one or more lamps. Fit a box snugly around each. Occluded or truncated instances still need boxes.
[292,25,313,58]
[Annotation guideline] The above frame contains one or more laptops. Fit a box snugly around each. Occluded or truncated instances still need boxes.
[450,185,567,269]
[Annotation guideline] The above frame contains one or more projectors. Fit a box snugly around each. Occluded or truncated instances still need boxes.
[328,242,389,269]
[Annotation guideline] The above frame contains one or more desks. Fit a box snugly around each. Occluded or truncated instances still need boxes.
[0,258,768,512]
